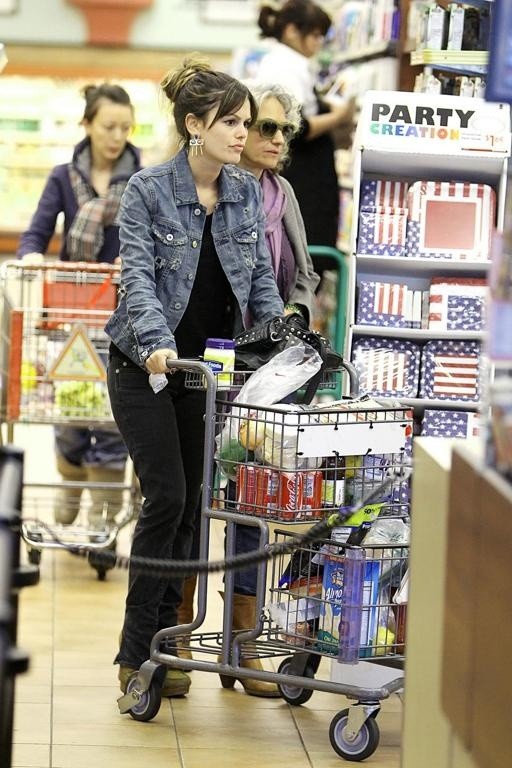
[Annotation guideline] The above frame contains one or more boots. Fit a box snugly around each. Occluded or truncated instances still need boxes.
[175,572,199,659]
[218,590,282,698]
[54,449,123,527]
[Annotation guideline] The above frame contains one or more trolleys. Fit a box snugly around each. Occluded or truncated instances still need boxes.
[0,260,140,581]
[117,352,416,762]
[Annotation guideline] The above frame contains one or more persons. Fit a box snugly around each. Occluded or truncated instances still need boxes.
[18,81,145,528]
[175,80,321,697]
[104,51,283,697]
[224,0,357,151]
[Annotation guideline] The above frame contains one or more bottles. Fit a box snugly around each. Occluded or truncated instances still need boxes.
[318,456,346,519]
[202,338,236,391]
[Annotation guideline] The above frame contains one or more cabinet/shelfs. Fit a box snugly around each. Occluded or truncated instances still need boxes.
[342,144,509,447]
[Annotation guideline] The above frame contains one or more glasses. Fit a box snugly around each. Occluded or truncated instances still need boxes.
[250,117,296,141]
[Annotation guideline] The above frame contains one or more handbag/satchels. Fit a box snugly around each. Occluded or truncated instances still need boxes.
[233,313,343,370]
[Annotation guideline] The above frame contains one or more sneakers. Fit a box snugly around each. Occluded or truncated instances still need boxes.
[118,658,191,697]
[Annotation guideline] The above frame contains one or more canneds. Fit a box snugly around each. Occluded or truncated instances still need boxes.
[234,461,323,521]
